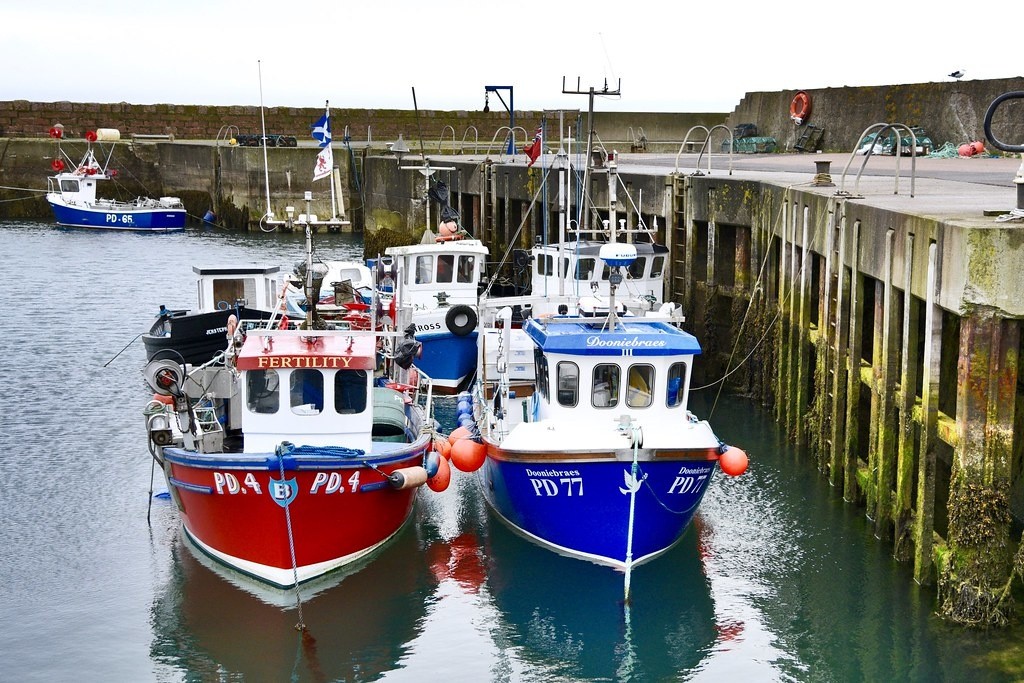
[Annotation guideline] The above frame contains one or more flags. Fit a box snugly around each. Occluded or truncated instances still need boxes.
[523,129,542,166]
[312,113,334,181]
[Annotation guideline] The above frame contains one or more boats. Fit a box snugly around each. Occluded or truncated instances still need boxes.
[143,58,439,592]
[45,172,188,231]
[471,76,723,573]
[284,162,490,395]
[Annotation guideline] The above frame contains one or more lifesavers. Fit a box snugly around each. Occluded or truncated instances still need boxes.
[790,92,809,119]
[445,305,477,337]
[435,235,463,242]
[227,314,238,337]
